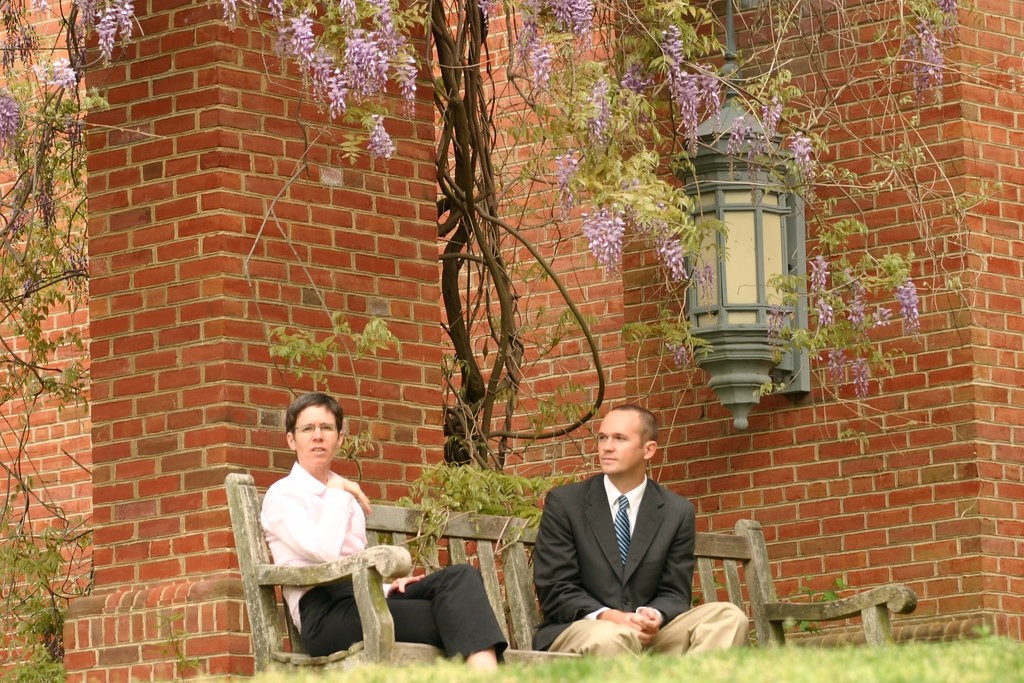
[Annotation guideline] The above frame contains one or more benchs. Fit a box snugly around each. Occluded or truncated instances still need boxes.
[226,471,917,673]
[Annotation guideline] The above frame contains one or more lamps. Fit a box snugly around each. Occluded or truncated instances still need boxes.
[669,0,811,429]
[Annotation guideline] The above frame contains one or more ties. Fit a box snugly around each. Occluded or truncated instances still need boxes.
[614,495,630,566]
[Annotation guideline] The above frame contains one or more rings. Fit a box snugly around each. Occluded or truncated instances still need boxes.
[361,492,366,496]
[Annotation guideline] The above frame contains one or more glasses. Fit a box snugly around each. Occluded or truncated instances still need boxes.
[295,423,338,433]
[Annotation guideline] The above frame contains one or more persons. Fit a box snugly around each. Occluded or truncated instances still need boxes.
[531,403,751,658]
[259,391,508,670]
